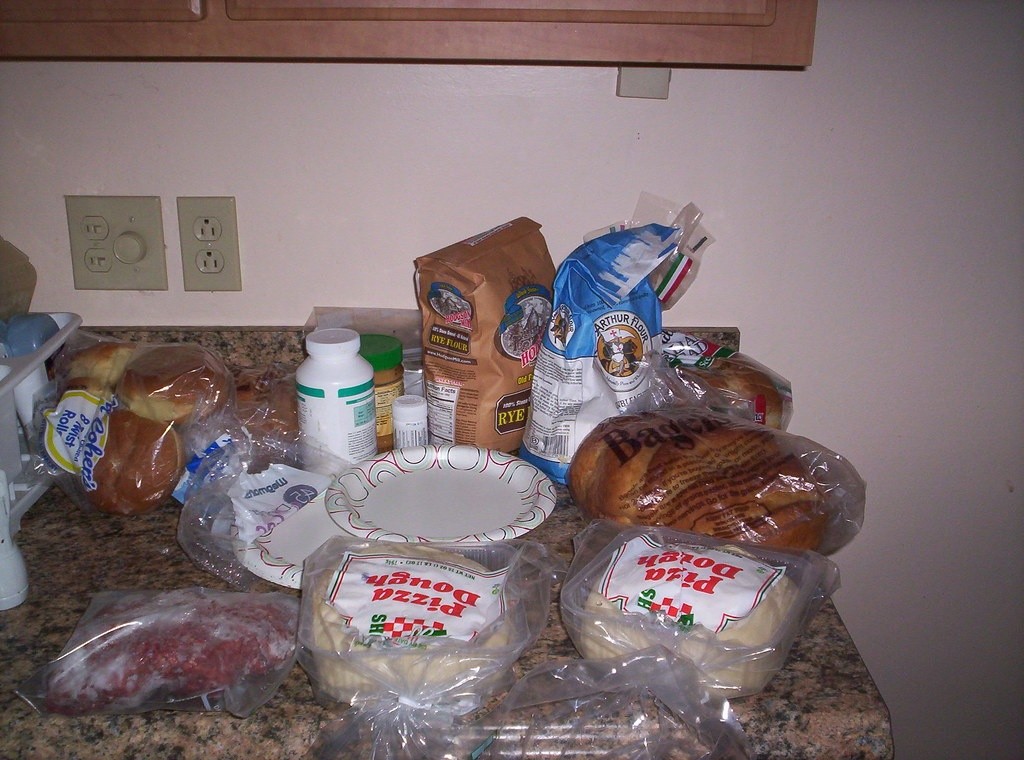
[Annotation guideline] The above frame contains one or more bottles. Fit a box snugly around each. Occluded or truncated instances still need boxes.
[357,334,406,454]
[294,328,378,477]
[390,394,430,450]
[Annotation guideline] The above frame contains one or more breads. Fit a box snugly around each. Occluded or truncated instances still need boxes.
[57,338,295,517]
[580,542,800,698]
[661,352,785,430]
[311,540,517,701]
[568,410,829,552]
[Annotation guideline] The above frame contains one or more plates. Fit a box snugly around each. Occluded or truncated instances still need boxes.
[234,487,357,590]
[325,444,557,546]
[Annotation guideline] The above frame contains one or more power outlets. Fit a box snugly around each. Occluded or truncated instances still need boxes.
[175,196,243,294]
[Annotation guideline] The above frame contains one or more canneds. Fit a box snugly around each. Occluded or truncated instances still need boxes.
[356,333,405,454]
